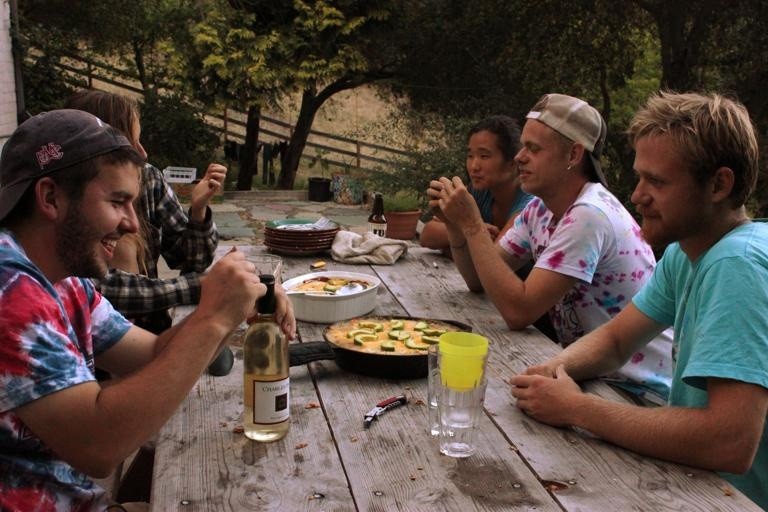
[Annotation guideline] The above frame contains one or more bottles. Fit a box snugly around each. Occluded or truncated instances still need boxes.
[368,192,387,238]
[243,274,290,443]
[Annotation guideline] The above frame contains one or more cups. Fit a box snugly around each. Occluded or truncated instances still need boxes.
[437,350,490,458]
[440,332,487,390]
[242,253,282,280]
[428,342,483,435]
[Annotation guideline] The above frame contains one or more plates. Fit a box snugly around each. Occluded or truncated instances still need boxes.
[263,219,341,256]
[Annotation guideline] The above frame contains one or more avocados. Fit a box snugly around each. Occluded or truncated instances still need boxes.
[207,344,235,377]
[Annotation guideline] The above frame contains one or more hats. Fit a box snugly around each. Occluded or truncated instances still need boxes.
[0,109,133,221]
[526,93,609,189]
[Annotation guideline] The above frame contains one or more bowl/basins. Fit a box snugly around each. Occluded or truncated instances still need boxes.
[282,271,381,322]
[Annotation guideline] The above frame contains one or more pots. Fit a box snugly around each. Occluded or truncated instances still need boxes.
[290,314,473,380]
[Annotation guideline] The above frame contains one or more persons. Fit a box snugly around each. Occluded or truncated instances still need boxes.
[41,89,228,334]
[418,111,563,344]
[425,90,678,422]
[0,108,297,510]
[508,88,768,511]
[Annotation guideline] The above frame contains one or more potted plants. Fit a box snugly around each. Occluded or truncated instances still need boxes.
[307,146,333,202]
[384,189,422,238]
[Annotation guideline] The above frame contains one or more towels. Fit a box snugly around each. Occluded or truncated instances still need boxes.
[331,229,407,265]
